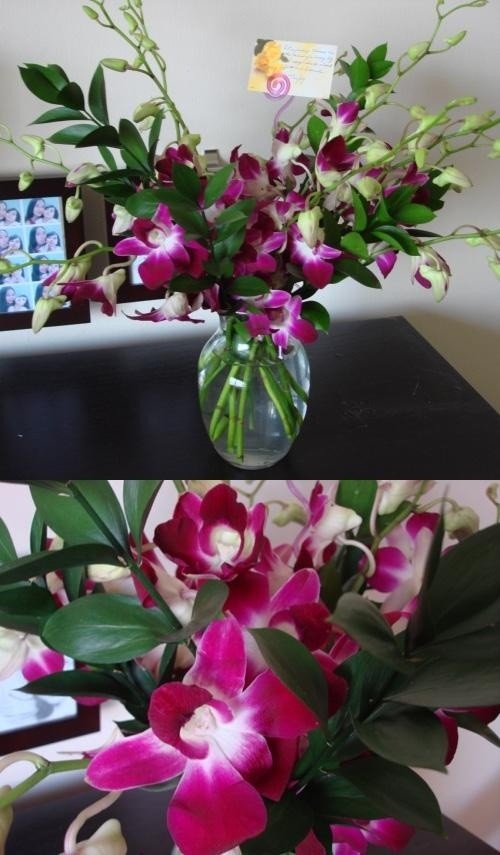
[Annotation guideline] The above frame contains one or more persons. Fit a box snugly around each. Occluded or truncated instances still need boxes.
[0,198,67,314]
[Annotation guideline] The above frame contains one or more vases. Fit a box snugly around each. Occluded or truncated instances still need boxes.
[198,320,312,470]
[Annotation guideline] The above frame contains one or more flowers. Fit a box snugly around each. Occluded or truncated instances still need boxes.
[0,479,500,854]
[1,0,500,460]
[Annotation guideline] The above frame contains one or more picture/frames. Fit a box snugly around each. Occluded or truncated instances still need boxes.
[102,168,173,304]
[2,176,92,331]
